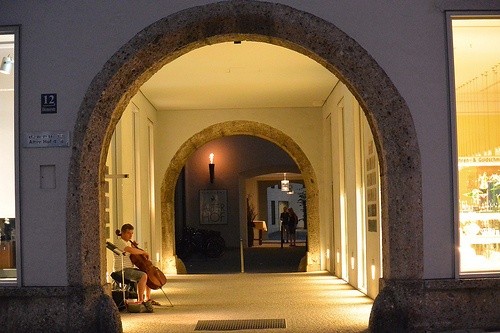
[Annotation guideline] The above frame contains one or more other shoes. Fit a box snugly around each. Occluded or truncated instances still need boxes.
[148,299,159,305]
[290,245,295,246]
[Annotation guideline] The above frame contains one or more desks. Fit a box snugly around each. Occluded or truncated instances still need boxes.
[253,220,267,246]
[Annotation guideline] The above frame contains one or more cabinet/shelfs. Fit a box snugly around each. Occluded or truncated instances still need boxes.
[458,148,500,274]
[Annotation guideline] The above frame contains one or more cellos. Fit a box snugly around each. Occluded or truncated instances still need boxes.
[115,229,174,307]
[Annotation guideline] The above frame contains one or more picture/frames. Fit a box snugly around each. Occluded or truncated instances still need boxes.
[199,188,228,225]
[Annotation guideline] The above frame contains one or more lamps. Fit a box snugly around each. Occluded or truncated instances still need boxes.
[286,182,295,195]
[208,153,217,184]
[0,54,13,75]
[281,173,289,191]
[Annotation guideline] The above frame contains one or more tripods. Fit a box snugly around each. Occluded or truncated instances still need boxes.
[106,241,129,311]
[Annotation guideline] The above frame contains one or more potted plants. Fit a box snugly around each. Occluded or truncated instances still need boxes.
[247,193,258,247]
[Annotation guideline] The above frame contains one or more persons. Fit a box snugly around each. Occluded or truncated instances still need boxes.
[280,207,299,247]
[112,224,162,309]
